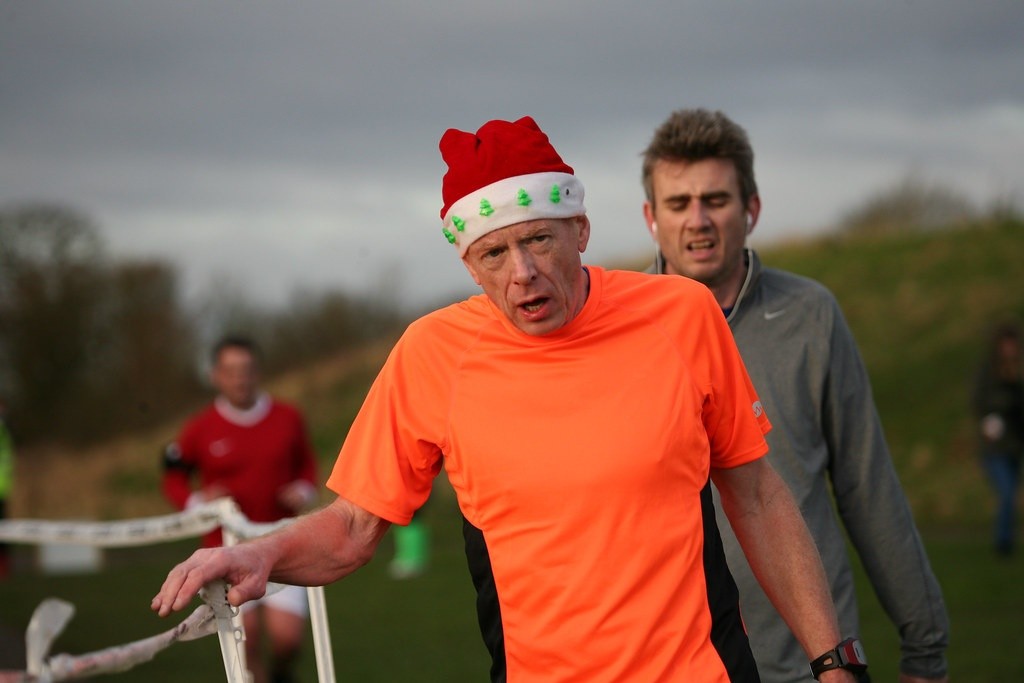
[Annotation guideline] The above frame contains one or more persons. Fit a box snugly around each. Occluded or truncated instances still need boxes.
[150,116,858,683]
[969,326,1024,555]
[639,109,951,683]
[158,334,319,683]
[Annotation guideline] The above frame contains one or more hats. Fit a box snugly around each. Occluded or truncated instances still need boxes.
[440,116,587,259]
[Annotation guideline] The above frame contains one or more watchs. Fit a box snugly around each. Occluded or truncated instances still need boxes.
[808,637,868,680]
